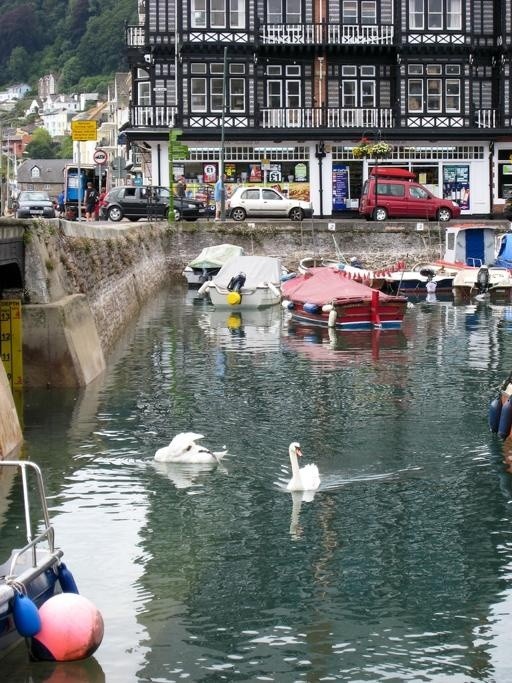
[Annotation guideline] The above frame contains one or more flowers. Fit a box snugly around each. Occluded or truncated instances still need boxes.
[367,140,393,155]
[351,137,374,157]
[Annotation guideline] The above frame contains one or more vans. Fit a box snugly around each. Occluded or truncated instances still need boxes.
[357,167,462,223]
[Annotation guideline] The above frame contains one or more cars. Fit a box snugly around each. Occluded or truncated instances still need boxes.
[504,188,511,222]
[14,190,55,219]
[225,187,315,222]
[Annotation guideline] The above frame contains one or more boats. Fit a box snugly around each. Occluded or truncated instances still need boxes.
[189,256,296,311]
[398,291,512,370]
[180,243,243,290]
[281,266,407,333]
[279,316,408,389]
[1,461,103,670]
[297,221,511,296]
[486,373,510,472]
[204,305,286,391]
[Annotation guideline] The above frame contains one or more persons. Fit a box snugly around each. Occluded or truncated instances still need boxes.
[176,173,186,196]
[59,190,66,214]
[98,187,109,217]
[214,174,229,220]
[84,182,99,223]
[52,201,57,209]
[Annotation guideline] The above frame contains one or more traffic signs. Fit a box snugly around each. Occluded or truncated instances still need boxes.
[72,131,97,141]
[168,153,187,161]
[70,122,97,131]
[168,146,188,153]
[170,128,183,135]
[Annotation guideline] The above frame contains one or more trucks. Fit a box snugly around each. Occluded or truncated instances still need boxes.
[61,163,96,218]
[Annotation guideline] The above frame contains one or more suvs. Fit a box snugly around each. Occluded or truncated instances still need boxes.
[99,186,199,222]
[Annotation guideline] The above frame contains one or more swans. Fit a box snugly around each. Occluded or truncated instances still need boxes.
[285,490,316,541]
[286,442,321,491]
[153,432,228,464]
